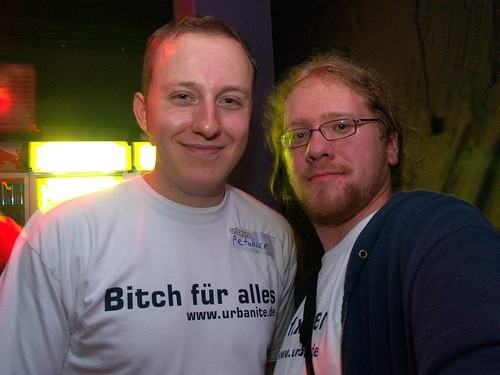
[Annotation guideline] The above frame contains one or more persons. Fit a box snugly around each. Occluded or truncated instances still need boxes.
[0,15,297,375]
[256,52,500,375]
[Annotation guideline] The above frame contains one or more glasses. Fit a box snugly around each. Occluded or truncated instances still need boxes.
[280,118,379,149]
